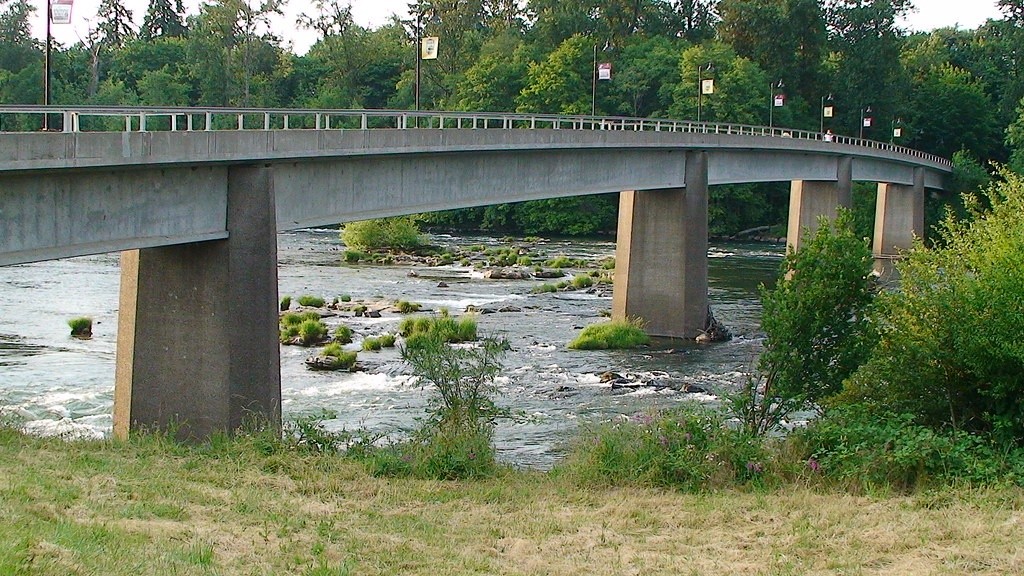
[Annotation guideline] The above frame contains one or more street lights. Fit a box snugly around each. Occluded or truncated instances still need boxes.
[591,37,615,115]
[914,128,925,150]
[890,116,904,143]
[860,104,873,139]
[415,6,443,128]
[770,78,785,133]
[820,92,834,132]
[697,61,715,134]
